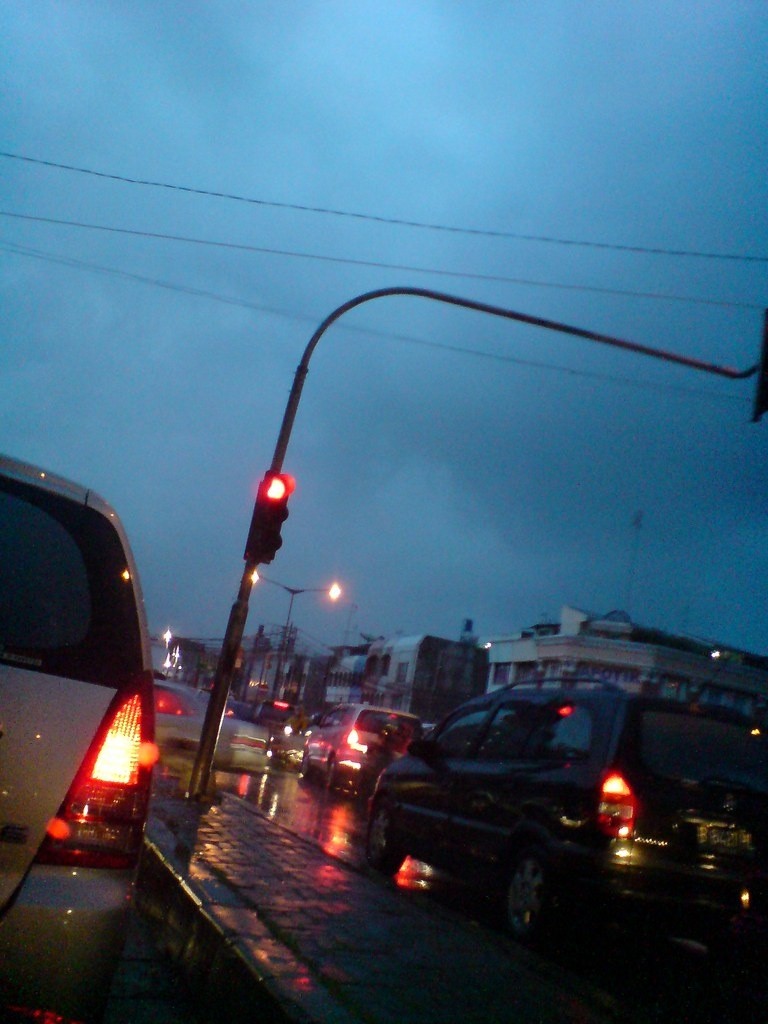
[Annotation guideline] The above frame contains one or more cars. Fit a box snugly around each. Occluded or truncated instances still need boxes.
[154,679,314,773]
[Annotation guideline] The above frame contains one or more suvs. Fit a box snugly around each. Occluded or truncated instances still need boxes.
[1,449,159,1024]
[361,676,768,965]
[301,702,423,799]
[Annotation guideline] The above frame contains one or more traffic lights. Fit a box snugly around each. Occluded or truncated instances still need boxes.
[256,469,295,508]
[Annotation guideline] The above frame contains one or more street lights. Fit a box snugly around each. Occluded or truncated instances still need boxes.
[251,569,342,754]
